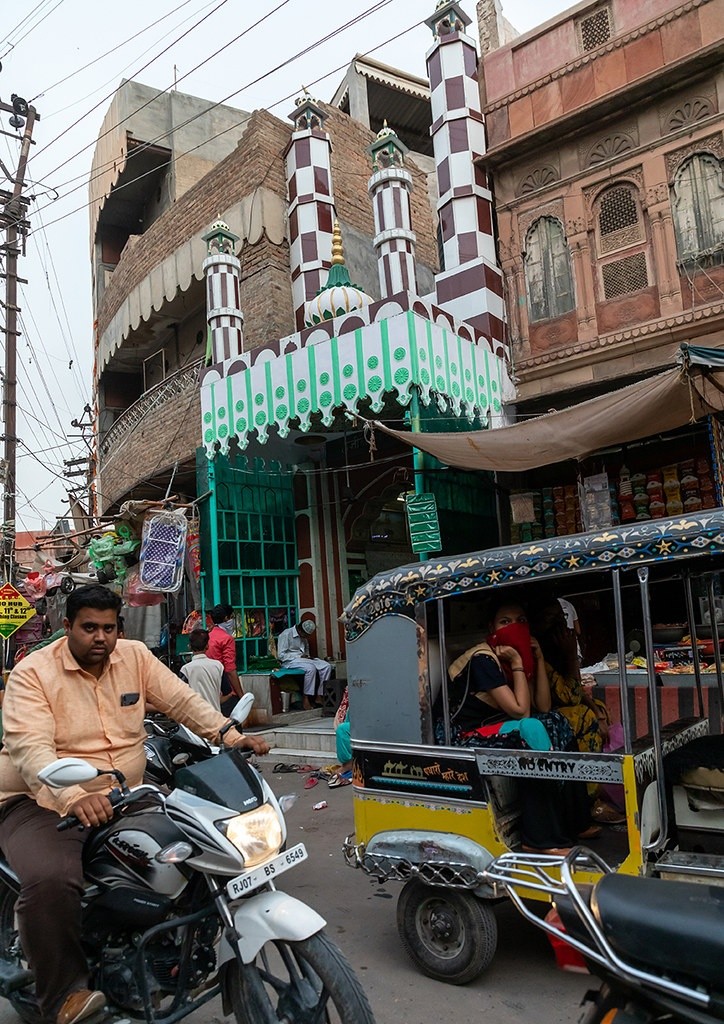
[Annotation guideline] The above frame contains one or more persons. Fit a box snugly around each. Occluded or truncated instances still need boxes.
[2,585,272,1024]
[178,628,235,756]
[523,591,631,824]
[548,586,584,671]
[205,601,251,735]
[334,686,355,777]
[278,620,334,713]
[436,593,605,856]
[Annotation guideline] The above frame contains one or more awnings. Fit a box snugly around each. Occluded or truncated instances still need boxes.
[338,342,724,475]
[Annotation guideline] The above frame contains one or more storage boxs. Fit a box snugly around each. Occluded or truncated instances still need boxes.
[593,668,652,688]
[696,639,724,655]
[657,669,724,687]
[696,623,724,638]
[650,627,688,643]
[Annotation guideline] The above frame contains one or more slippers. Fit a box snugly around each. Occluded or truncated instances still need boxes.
[320,772,329,781]
[279,764,300,773]
[328,775,353,789]
[306,765,322,771]
[296,764,312,773]
[310,771,320,780]
[327,773,341,784]
[272,762,289,773]
[303,777,318,789]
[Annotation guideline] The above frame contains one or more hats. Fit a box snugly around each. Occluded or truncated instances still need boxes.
[302,619,315,635]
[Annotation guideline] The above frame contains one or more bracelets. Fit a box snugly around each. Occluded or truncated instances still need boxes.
[512,668,527,674]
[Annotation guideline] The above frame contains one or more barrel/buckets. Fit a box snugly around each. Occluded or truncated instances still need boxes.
[280,691,291,713]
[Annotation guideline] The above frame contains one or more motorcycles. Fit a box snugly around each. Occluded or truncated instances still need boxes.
[0,691,379,1024]
[138,711,202,795]
[476,842,724,1023]
[341,507,724,986]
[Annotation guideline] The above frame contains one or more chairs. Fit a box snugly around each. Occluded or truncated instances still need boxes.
[238,664,337,723]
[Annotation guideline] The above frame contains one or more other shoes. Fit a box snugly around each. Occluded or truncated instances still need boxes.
[521,844,573,855]
[577,824,603,838]
[590,798,627,823]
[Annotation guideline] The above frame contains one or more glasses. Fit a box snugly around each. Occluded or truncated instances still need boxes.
[531,612,568,624]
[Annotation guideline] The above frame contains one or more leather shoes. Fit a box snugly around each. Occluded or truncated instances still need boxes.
[53,985,107,1024]
